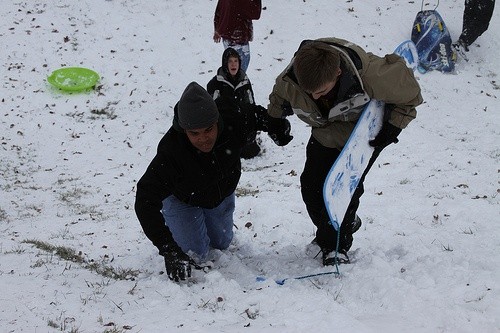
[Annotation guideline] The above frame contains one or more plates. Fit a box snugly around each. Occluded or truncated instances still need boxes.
[47,67,99,90]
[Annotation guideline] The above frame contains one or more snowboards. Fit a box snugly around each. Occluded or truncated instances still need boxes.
[322,40,420,231]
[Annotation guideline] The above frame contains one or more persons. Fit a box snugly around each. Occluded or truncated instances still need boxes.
[451,0,495,62]
[213,0,262,72]
[134,82,292,286]
[207,47,260,158]
[268,37,423,268]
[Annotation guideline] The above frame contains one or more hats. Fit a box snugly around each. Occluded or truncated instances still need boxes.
[176,81,218,131]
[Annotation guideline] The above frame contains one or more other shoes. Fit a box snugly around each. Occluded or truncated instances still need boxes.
[341,213,364,237]
[449,40,471,63]
[320,245,351,267]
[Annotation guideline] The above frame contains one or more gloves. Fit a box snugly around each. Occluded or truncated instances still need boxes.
[264,116,296,148]
[158,238,203,284]
[367,122,403,150]
